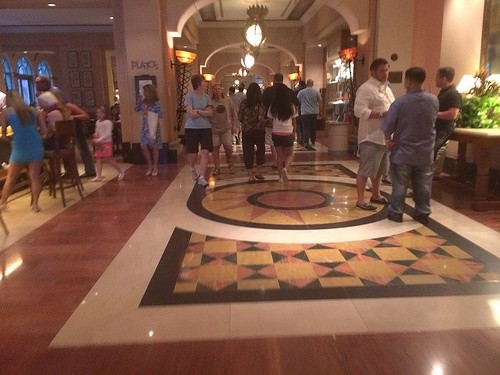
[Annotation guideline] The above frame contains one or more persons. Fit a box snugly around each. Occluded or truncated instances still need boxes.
[87,104,125,183]
[109,103,124,155]
[354,58,401,211]
[364,147,392,192]
[238,72,301,184]
[385,66,440,223]
[404,66,462,197]
[207,82,235,177]
[133,83,164,177]
[294,78,322,151]
[0,90,47,214]
[228,82,247,145]
[33,75,97,185]
[185,73,214,187]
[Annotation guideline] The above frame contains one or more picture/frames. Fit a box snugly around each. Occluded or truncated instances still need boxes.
[135,76,157,104]
[68,52,95,107]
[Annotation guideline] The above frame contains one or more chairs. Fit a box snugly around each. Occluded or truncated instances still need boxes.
[46,120,86,209]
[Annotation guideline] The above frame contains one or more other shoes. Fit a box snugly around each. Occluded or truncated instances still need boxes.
[255,174,265,179]
[89,177,102,182]
[118,170,125,181]
[279,178,283,183]
[414,213,428,223]
[212,168,220,174]
[312,141,315,144]
[406,191,414,196]
[152,172,158,176]
[230,168,235,173]
[63,172,73,177]
[305,143,309,146]
[0,202,6,209]
[356,200,377,210]
[145,171,151,175]
[388,211,403,222]
[282,169,288,182]
[371,194,389,204]
[80,171,96,177]
[271,164,277,169]
[249,178,256,183]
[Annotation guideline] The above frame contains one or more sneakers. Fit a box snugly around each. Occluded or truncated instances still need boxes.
[192,170,199,180]
[198,177,209,187]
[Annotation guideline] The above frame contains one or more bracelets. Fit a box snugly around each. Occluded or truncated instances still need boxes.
[380,111,383,119]
[197,109,200,113]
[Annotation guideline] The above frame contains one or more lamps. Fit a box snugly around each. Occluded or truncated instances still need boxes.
[239,3,268,77]
[170,49,198,68]
[339,47,365,64]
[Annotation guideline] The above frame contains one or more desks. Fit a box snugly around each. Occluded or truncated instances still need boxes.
[446,128,500,211]
